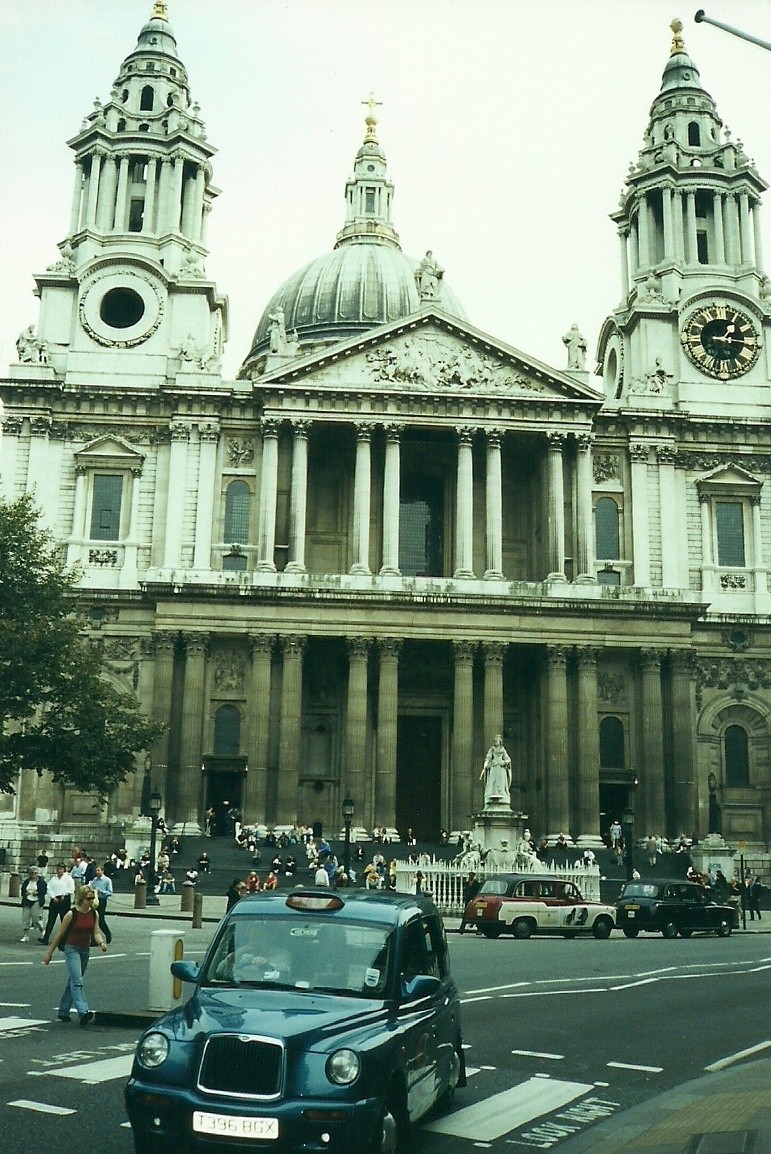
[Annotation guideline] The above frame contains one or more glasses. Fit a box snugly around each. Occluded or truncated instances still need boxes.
[84,897,95,900]
[32,872,37,874]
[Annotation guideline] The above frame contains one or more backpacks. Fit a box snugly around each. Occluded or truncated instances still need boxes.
[57,906,96,952]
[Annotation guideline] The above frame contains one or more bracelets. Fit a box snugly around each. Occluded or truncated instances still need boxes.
[100,942,104,947]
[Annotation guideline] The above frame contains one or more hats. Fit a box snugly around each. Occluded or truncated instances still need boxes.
[159,849,169,854]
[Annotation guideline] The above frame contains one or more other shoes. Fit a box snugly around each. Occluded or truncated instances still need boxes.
[41,930,46,938]
[749,916,761,921]
[273,869,280,874]
[285,871,293,877]
[29,927,34,930]
[19,934,29,942]
[58,1014,71,1022]
[246,890,258,894]
[304,841,313,845]
[89,942,98,947]
[37,937,48,945]
[106,932,111,944]
[80,1011,94,1026]
[455,929,481,935]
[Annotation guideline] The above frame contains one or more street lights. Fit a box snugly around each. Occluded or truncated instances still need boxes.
[133,750,155,827]
[621,808,635,882]
[707,772,725,845]
[145,791,162,905]
[341,799,355,888]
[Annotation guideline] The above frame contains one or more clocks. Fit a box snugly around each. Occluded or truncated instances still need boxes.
[680,304,760,378]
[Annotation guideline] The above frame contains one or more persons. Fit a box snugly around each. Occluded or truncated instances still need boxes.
[644,831,698,854]
[226,822,450,916]
[360,339,494,389]
[456,831,470,858]
[43,886,108,1026]
[456,872,483,935]
[609,819,626,865]
[20,835,181,945]
[485,735,511,796]
[421,251,442,298]
[158,818,168,834]
[205,806,242,837]
[687,866,761,920]
[562,323,587,369]
[632,869,640,880]
[574,848,596,869]
[215,923,293,982]
[646,835,656,867]
[520,829,533,858]
[178,332,214,371]
[266,307,287,352]
[17,326,49,364]
[645,357,667,393]
[186,852,211,885]
[529,833,567,852]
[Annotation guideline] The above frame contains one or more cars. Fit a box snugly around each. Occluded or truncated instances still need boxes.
[465,875,617,939]
[122,886,468,1152]
[614,879,736,940]
